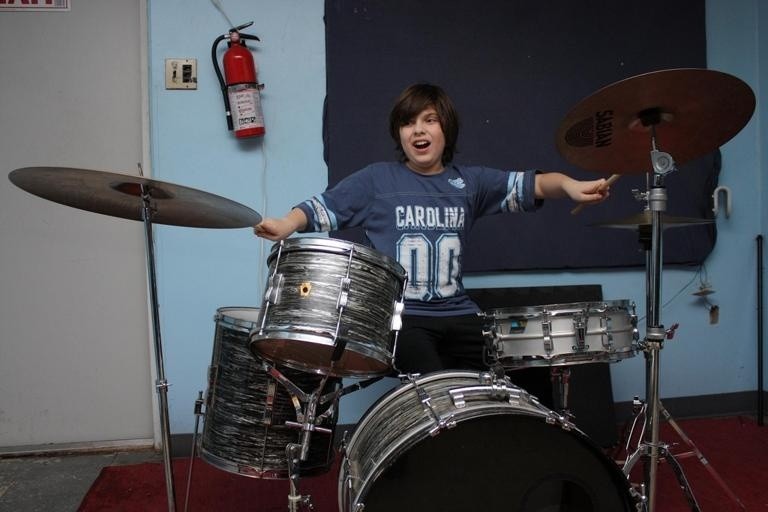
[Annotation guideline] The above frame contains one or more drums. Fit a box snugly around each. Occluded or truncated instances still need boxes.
[196,306,334,482]
[483,298,639,371]
[337,369,646,512]
[248,238,407,375]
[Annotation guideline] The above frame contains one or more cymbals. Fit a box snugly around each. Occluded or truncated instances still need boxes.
[553,68,757,175]
[8,166,262,228]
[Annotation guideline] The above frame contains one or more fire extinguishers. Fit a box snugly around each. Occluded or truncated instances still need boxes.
[211,21,266,138]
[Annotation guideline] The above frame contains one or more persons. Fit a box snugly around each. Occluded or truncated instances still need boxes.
[251,84,611,377]
